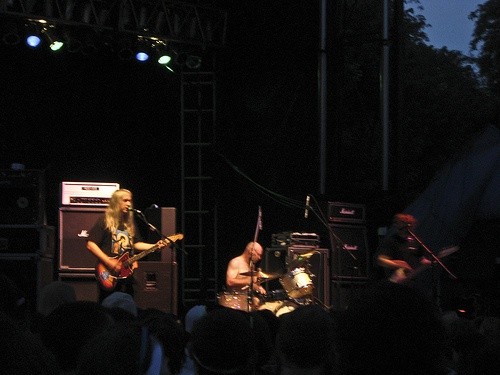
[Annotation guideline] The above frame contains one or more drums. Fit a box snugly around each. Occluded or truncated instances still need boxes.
[218,291,248,311]
[257,298,297,315]
[282,268,317,299]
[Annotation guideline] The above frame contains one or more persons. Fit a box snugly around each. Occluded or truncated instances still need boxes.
[226,242,277,290]
[280,250,314,304]
[0,274,500,375]
[86,188,167,304]
[369,213,429,280]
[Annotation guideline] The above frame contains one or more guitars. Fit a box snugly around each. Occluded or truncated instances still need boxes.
[383,244,459,284]
[95,233,184,291]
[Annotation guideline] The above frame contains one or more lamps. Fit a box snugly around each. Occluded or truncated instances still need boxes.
[166,44,189,65]
[114,36,135,62]
[183,48,202,69]
[39,23,65,52]
[96,33,116,56]
[59,26,82,54]
[22,21,43,49]
[135,41,154,63]
[76,29,98,56]
[151,39,173,65]
[1,17,22,46]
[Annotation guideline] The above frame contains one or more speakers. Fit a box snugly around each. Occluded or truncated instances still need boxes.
[57,274,107,304]
[134,208,177,261]
[326,227,371,279]
[266,246,328,308]
[59,206,113,272]
[333,282,369,306]
[131,260,177,320]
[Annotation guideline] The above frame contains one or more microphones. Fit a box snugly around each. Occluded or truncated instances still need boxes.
[128,207,142,214]
[304,195,310,218]
[258,207,262,231]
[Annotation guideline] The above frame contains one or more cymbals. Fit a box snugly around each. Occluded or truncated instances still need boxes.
[240,272,284,278]
[289,250,316,269]
